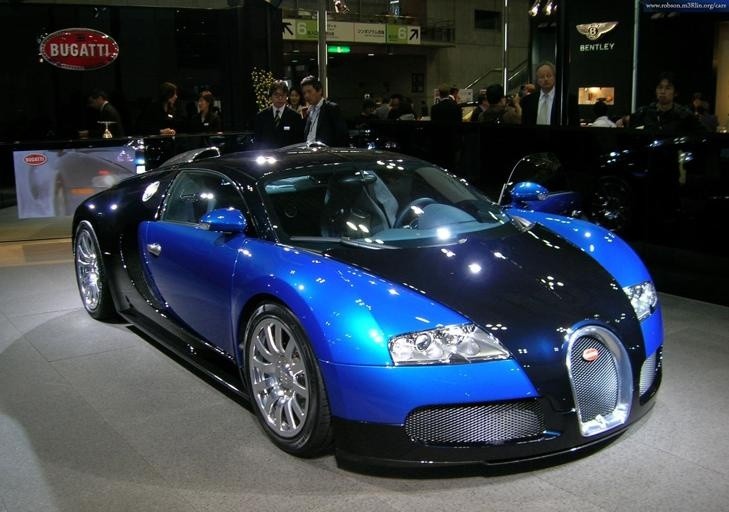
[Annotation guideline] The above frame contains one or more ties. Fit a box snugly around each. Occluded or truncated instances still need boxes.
[536,95,550,125]
[273,110,281,128]
[304,108,315,130]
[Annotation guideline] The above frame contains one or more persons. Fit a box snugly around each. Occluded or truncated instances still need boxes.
[133,82,188,133]
[285,88,305,120]
[254,79,306,146]
[351,56,714,157]
[300,75,346,150]
[84,86,123,138]
[192,88,223,131]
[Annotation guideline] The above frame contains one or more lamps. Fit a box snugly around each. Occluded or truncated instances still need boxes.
[527,0,558,16]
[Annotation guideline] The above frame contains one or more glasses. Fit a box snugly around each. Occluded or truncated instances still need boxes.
[272,93,286,101]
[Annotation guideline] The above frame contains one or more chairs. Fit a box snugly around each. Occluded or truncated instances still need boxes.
[322,171,391,241]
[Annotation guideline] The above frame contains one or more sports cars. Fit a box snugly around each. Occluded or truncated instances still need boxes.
[69,139,665,474]
[28,145,147,215]
